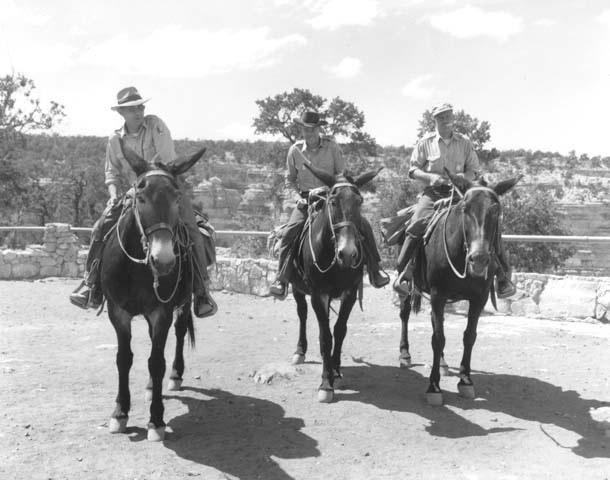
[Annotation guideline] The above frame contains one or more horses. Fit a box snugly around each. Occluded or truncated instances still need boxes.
[100,137,206,441]
[398,167,525,407]
[289,163,386,404]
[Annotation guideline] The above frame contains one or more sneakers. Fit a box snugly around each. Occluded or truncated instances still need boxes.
[198,297,212,315]
[401,278,411,290]
[270,280,284,295]
[501,282,512,294]
[374,272,387,285]
[71,287,89,304]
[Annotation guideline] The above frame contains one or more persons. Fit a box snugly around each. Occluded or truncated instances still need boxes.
[392,101,517,299]
[68,86,219,318]
[268,111,390,302]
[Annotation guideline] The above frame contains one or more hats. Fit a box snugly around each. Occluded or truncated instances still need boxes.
[431,103,452,117]
[293,111,327,128]
[112,87,149,108]
